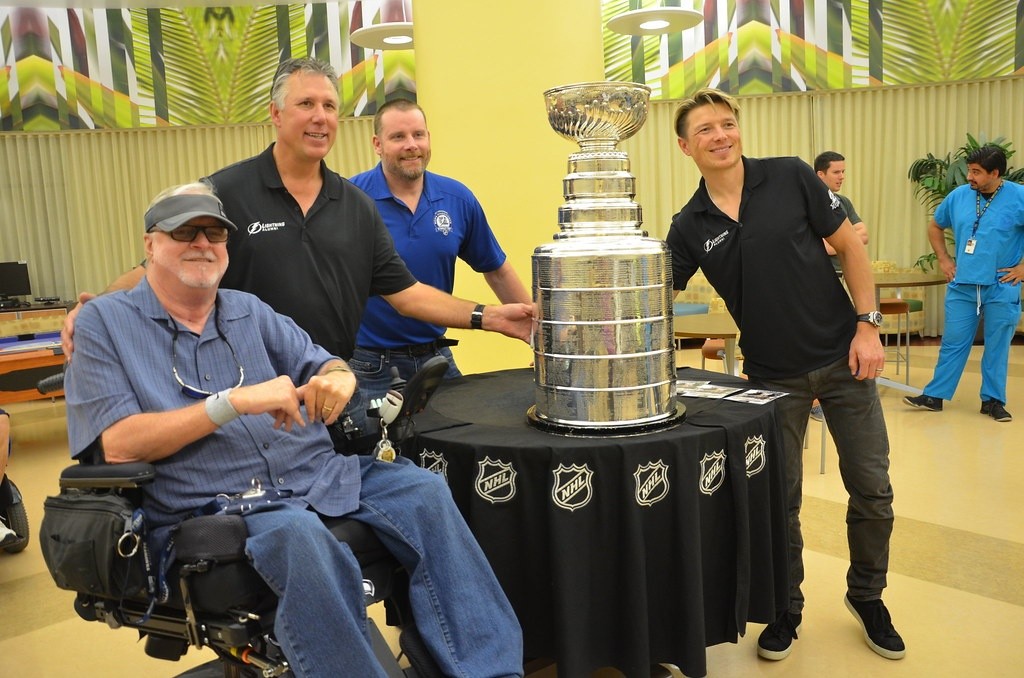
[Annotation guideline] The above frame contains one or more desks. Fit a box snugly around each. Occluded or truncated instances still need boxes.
[874,273,948,362]
[674,313,741,376]
[385,369,790,678]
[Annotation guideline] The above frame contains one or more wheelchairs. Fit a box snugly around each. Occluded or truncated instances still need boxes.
[36,362,396,678]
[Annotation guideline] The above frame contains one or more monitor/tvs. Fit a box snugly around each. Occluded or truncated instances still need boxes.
[0,261,32,302]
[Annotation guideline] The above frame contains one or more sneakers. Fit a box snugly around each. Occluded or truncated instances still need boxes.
[903,394,943,410]
[0,514,14,545]
[757,610,802,659]
[981,397,1013,422]
[846,591,905,659]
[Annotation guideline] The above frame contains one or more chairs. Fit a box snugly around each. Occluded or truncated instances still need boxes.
[702,297,744,378]
[878,270,922,388]
[34,358,448,678]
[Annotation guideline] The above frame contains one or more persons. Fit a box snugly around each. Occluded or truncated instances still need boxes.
[60,57,531,454]
[903,145,1024,422]
[809,151,869,422]
[663,89,905,660]
[65,184,524,678]
[0,409,18,545]
[344,99,531,445]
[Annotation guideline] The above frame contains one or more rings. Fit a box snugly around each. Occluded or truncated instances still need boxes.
[323,404,333,411]
[876,368,884,371]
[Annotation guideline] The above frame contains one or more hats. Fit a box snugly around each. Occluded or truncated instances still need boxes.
[143,195,237,234]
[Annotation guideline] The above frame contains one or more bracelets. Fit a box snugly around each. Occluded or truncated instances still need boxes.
[205,387,241,427]
[322,365,352,374]
[470,304,485,330]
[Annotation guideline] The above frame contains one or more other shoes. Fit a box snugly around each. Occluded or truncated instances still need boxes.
[810,406,825,420]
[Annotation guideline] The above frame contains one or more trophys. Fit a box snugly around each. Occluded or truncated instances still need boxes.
[527,81,687,437]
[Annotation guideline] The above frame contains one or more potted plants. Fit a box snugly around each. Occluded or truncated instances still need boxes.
[908,133,1024,344]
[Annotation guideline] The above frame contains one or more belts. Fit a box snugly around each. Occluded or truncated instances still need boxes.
[362,335,460,354]
[835,272,844,278]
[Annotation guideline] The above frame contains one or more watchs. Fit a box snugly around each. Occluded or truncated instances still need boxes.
[855,311,882,327]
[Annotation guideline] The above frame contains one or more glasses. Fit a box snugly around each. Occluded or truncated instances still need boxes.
[150,224,231,244]
